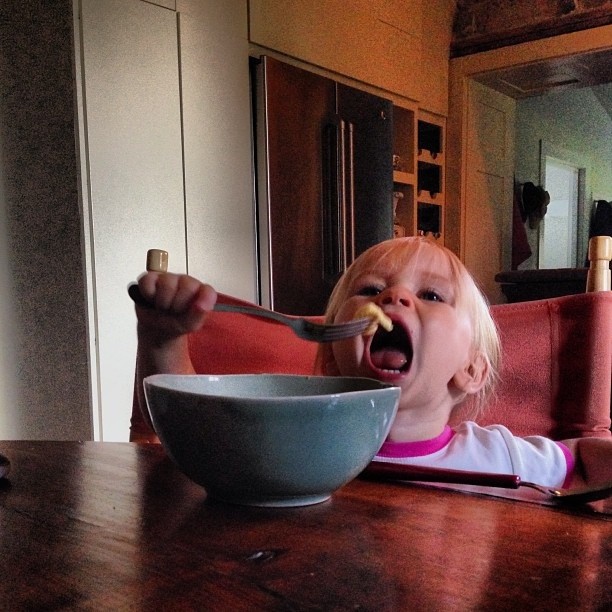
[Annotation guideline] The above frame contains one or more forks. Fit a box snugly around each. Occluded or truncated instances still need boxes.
[128,281,373,342]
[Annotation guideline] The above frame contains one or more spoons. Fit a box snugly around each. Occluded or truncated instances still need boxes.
[354,460,612,504]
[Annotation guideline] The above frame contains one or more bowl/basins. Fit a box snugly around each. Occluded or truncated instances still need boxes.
[143,373,401,508]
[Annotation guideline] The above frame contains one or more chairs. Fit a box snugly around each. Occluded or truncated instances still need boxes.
[128,283,611,526]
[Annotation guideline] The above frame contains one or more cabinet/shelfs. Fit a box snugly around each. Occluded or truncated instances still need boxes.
[1,0,261,442]
[393,94,445,250]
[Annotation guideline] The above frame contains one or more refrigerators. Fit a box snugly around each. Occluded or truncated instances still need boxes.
[251,55,393,316]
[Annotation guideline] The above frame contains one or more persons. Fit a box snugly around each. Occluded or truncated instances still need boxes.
[130,235,611,502]
